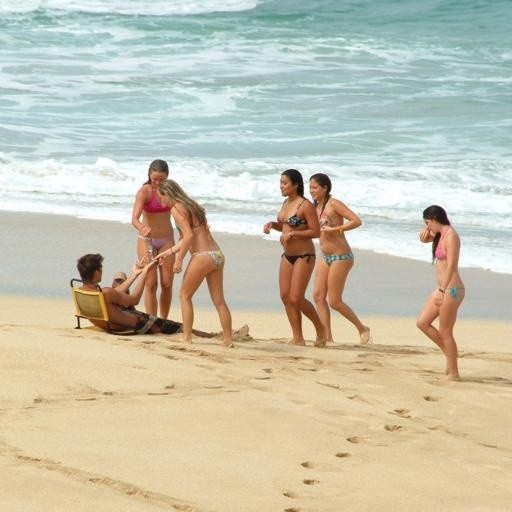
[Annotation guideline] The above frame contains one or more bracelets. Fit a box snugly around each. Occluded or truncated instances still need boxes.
[438,287,445,293]
[336,224,343,234]
[171,247,175,255]
[287,230,296,240]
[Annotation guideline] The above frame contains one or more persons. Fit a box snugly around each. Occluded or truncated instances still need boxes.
[77,253,249,341]
[416,205,465,382]
[153,179,235,345]
[132,160,176,320]
[263,169,328,348]
[305,172,370,345]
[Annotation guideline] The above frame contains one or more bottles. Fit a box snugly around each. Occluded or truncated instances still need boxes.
[146,235,154,259]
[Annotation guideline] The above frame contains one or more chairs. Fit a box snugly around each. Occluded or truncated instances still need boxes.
[71,279,137,334]
